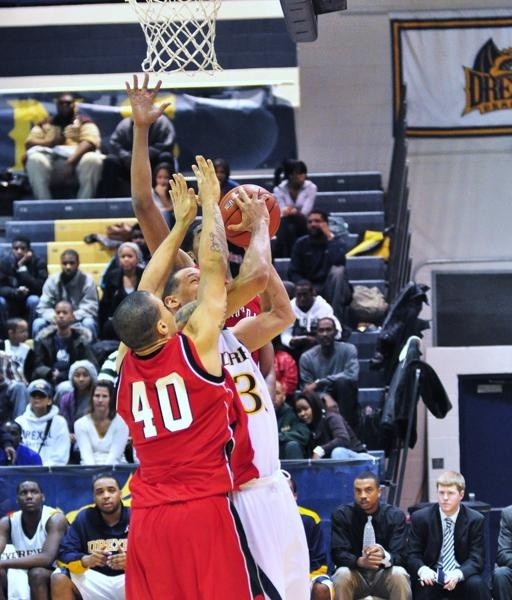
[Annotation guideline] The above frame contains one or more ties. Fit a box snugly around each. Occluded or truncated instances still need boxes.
[362,516,375,549]
[442,520,456,571]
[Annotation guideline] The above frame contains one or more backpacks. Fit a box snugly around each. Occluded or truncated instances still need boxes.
[356,403,388,451]
[350,285,387,322]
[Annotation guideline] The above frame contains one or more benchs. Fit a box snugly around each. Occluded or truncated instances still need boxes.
[1,84,151,472]
[186,170,391,471]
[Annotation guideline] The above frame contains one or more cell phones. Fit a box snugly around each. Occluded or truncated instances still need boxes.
[437,569,447,585]
[105,551,119,569]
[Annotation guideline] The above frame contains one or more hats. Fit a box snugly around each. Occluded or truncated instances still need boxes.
[29,379,51,397]
[67,360,97,383]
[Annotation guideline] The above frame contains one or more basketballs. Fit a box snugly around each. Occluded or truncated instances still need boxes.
[219,184,282,248]
